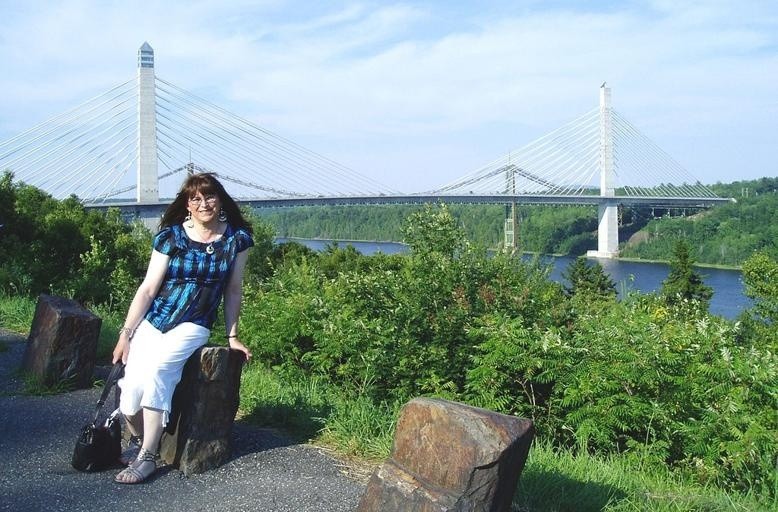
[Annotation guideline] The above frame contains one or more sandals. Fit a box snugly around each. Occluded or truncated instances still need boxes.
[114,434,156,483]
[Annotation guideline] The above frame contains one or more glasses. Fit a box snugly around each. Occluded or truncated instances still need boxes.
[190,195,215,204]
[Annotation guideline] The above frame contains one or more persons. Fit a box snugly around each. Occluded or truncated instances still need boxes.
[194,221,220,254]
[111,173,255,484]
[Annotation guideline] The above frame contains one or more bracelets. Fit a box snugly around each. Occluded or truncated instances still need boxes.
[224,335,239,338]
[119,327,134,338]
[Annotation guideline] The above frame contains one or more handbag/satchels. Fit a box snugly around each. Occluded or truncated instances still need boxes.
[72,418,121,471]
